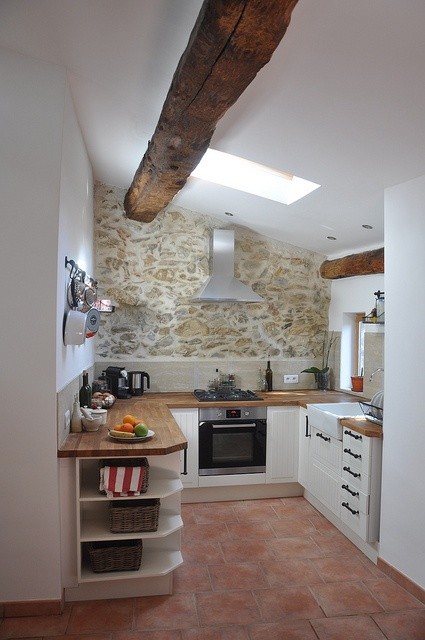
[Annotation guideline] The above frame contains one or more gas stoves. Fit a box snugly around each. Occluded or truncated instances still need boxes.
[192,389,264,402]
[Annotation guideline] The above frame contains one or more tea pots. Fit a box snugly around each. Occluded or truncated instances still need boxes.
[98,372,112,389]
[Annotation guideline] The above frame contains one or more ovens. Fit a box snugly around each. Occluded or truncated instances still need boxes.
[198,419,267,476]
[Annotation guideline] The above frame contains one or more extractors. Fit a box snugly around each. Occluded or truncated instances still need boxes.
[191,229,264,303]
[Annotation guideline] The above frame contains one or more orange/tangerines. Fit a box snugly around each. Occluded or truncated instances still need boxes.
[133,424,149,438]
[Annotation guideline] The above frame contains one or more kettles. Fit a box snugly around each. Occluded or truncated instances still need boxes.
[127,371,150,396]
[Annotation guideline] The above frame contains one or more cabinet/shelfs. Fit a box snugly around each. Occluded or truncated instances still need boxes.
[298,406,310,491]
[340,425,372,542]
[168,407,199,488]
[266,406,299,483]
[77,455,184,584]
[307,406,342,518]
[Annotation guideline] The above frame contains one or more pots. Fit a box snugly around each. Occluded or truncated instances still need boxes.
[63,278,88,346]
[77,278,96,313]
[85,306,100,338]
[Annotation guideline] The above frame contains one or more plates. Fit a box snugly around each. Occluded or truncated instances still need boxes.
[371,391,382,417]
[379,393,383,419]
[372,392,383,418]
[107,429,155,441]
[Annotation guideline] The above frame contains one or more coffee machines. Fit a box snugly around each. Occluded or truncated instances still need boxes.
[102,366,135,399]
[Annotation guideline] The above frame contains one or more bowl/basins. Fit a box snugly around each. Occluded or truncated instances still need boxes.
[82,415,102,431]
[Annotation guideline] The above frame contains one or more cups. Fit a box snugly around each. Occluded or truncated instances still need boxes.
[92,406,108,425]
[81,405,93,418]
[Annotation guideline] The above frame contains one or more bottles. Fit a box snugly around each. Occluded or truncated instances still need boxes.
[86,373,91,408]
[71,404,82,433]
[75,393,81,411]
[260,375,268,392]
[266,355,272,391]
[257,368,262,392]
[79,370,89,408]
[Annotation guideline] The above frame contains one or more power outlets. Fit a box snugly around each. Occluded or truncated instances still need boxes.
[284,375,298,383]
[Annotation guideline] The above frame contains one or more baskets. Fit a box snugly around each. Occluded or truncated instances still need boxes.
[110,498,160,533]
[88,539,142,572]
[98,458,150,494]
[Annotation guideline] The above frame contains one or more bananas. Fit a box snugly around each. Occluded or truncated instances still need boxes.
[107,429,135,439]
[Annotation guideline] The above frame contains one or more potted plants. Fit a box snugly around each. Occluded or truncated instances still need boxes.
[301,330,339,392]
[351,367,364,392]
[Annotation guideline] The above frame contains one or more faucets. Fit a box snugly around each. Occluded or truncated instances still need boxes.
[369,368,384,383]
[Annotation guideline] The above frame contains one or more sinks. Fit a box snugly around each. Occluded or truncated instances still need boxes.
[306,402,371,417]
